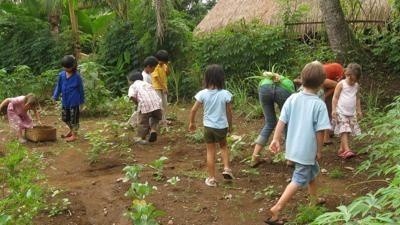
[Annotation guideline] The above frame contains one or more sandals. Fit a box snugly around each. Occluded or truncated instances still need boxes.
[159,120,173,126]
[309,197,327,208]
[263,216,285,225]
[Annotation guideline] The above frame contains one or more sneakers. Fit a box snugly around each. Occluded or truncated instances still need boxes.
[343,150,356,160]
[66,134,78,142]
[338,149,351,157]
[61,130,72,138]
[250,154,267,168]
[205,176,217,187]
[17,138,28,144]
[222,168,235,180]
[149,132,158,143]
[322,139,334,146]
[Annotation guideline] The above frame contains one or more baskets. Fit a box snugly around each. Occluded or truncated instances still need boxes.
[24,125,57,143]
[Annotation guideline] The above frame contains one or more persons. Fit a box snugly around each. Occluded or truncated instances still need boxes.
[126,69,164,147]
[188,63,235,189]
[330,62,364,161]
[151,49,173,126]
[126,56,160,132]
[0,93,41,146]
[294,61,347,145]
[263,60,332,225]
[249,69,297,170]
[50,54,85,143]
